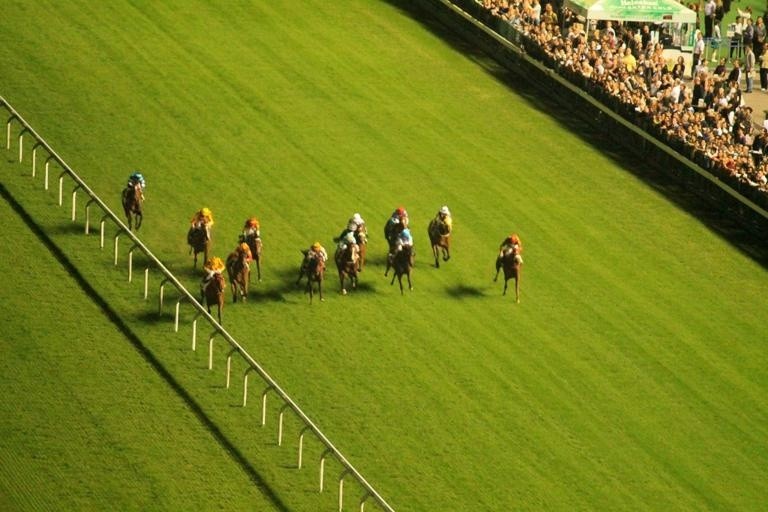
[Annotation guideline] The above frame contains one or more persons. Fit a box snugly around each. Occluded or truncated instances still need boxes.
[480,0,767,195]
[304,242,328,265]
[203,256,225,282]
[385,207,411,227]
[350,213,365,241]
[226,243,252,267]
[190,206,213,241]
[335,222,360,259]
[432,204,453,234]
[130,172,145,196]
[392,218,413,252]
[498,232,522,260]
[241,217,261,238]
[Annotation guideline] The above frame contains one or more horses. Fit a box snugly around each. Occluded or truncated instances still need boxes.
[295,249,326,304]
[385,243,414,294]
[427,218,451,268]
[385,215,407,248]
[187,221,264,325]
[333,223,367,271]
[335,242,359,295]
[121,180,143,231]
[494,247,521,304]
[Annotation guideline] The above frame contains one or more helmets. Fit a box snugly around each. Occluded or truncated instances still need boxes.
[401,229,410,239]
[240,242,249,252]
[440,205,451,216]
[313,241,320,248]
[510,233,520,244]
[246,218,257,226]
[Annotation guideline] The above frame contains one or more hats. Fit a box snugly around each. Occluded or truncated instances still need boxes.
[130,172,144,182]
[352,212,366,226]
[202,207,210,217]
